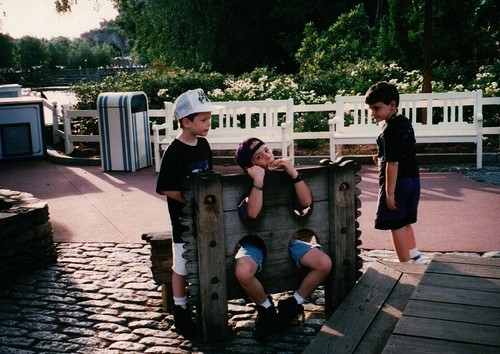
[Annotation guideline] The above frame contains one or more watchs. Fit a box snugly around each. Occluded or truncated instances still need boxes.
[292,172,304,184]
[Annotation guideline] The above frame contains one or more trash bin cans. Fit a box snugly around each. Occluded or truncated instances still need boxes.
[0,97,46,162]
[97,92,153,172]
[0,84,22,97]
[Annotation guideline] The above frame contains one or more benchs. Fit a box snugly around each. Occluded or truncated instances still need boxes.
[302,259,430,354]
[329,90,482,169]
[141,231,174,313]
[152,97,294,172]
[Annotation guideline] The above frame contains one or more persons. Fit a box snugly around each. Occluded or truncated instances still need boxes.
[365,81,423,262]
[156,88,214,330]
[234,137,332,341]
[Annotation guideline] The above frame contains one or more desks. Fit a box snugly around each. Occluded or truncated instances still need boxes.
[381,253,500,354]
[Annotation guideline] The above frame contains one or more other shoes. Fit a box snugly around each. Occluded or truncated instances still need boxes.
[276,295,306,339]
[253,293,275,343]
[173,300,190,330]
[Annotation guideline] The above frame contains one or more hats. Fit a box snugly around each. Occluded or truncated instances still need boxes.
[173,87,212,120]
[234,137,265,173]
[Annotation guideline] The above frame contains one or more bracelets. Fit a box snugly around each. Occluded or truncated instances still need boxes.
[252,184,264,190]
